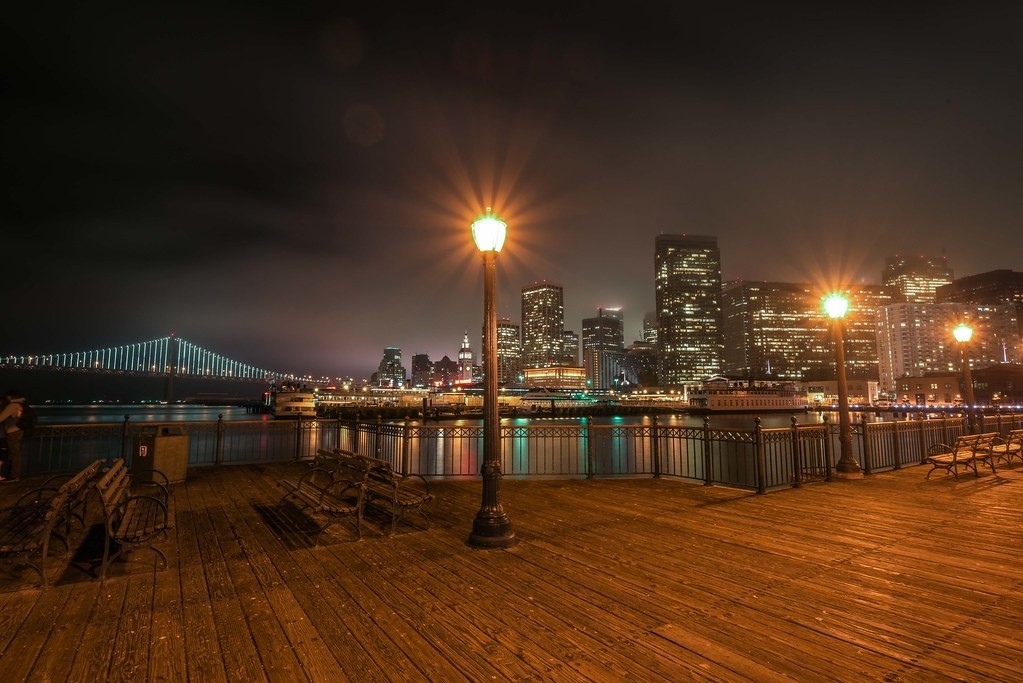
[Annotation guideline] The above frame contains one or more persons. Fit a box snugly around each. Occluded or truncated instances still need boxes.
[0,389,25,483]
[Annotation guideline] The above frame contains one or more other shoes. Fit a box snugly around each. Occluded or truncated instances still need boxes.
[1,476,20,484]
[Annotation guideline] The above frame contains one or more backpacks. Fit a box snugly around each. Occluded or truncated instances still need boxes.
[9,402,37,430]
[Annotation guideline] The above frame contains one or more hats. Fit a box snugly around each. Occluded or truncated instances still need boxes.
[7,390,21,395]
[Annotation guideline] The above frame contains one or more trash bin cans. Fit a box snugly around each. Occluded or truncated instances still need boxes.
[132,424,190,487]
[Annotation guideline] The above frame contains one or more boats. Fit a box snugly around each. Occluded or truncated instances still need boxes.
[264,379,317,420]
[683,375,813,415]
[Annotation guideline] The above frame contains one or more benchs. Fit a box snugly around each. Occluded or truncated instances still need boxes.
[274,448,433,548]
[0,456,169,584]
[923,430,1023,483]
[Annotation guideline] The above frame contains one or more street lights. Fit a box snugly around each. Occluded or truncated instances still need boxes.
[822,293,861,473]
[469,207,517,547]
[952,322,985,452]
